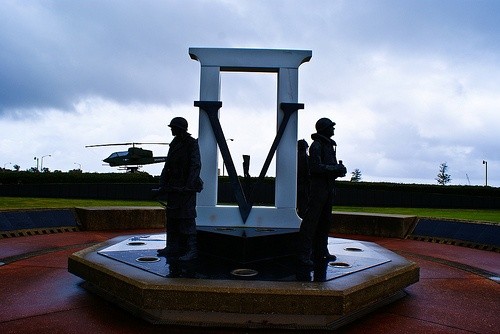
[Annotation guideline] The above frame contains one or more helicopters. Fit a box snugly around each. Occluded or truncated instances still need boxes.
[85,142,172,170]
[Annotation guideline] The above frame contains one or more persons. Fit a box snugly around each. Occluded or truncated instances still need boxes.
[156,117,201,260]
[299,118,347,266]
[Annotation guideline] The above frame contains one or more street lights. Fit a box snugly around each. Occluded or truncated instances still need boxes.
[41,154,51,172]
[482,160,490,186]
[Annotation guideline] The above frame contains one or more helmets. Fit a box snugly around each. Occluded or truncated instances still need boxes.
[298,140,308,150]
[168,117,188,128]
[315,118,336,131]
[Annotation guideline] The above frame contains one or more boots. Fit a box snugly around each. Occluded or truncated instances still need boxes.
[296,238,314,266]
[177,231,199,262]
[157,232,181,255]
[322,242,336,261]
